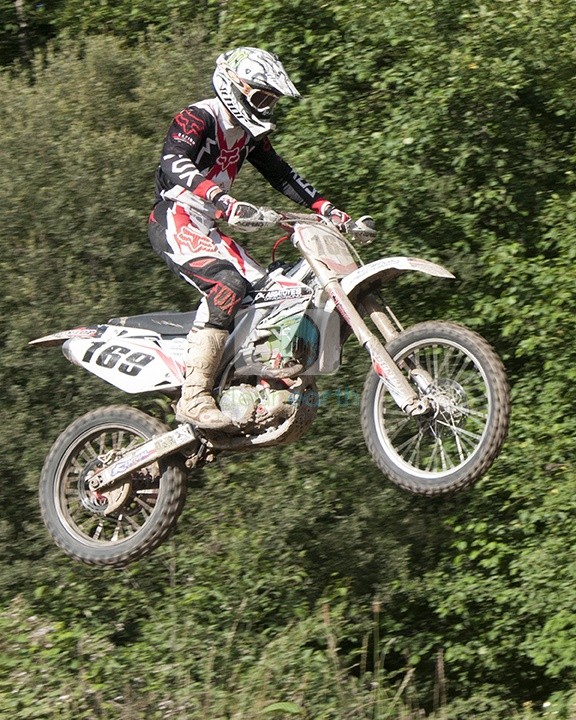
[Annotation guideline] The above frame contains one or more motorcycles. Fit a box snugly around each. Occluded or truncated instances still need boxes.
[27,201,513,568]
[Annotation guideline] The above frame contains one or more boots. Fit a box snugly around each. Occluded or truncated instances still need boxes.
[174,326,231,428]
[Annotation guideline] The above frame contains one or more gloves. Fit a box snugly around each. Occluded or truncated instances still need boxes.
[322,203,351,232]
[212,193,237,220]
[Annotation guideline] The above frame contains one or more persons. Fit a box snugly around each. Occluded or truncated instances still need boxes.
[150,48,349,429]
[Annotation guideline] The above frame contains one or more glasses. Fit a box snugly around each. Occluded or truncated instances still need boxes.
[246,88,279,114]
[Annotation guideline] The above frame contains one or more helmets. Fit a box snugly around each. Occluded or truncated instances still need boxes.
[212,46,301,138]
